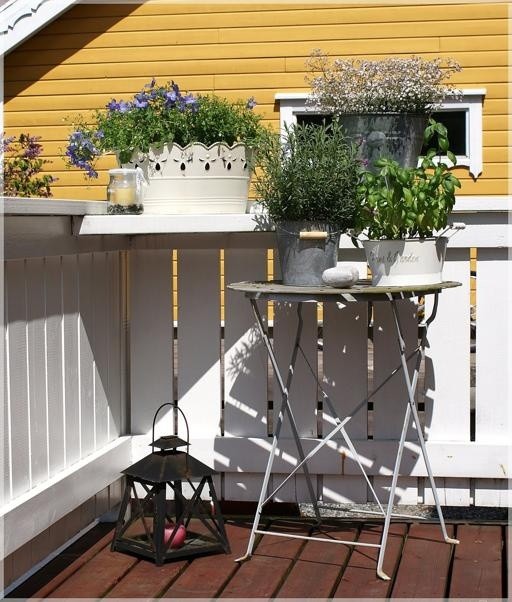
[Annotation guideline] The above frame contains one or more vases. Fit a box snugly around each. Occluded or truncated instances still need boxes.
[339,110,432,175]
[114,140,252,214]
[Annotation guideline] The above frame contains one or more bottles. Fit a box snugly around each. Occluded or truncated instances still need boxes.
[106,166,143,214]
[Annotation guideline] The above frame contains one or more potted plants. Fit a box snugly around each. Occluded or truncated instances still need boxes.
[237,116,461,286]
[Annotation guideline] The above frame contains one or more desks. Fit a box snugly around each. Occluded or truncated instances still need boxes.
[226,280,463,581]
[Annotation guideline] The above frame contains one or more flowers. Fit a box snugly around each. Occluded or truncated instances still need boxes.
[56,76,266,184]
[0,130,59,198]
[300,47,464,115]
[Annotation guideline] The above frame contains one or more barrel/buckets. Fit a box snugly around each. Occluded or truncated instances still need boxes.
[272,216,344,286]
[332,108,431,181]
